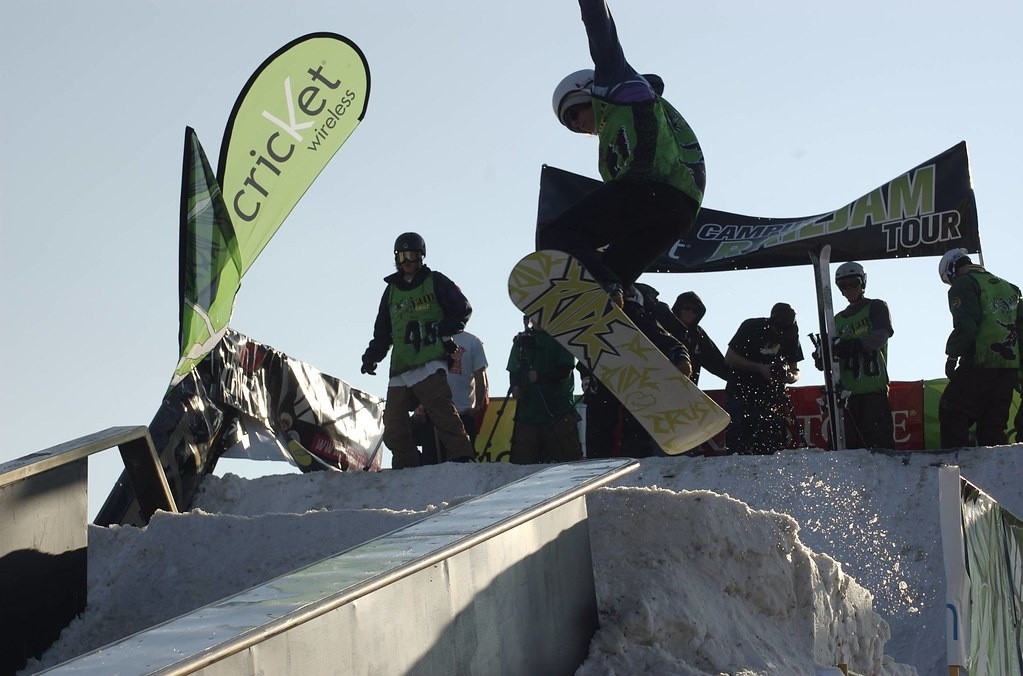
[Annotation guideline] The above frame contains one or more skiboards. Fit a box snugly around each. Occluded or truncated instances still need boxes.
[806,244,847,452]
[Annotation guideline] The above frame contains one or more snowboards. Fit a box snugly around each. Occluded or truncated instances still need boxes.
[507,249,732,456]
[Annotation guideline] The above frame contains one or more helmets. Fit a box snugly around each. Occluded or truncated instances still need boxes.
[835,262,866,289]
[394,232,425,255]
[553,69,594,125]
[939,249,966,282]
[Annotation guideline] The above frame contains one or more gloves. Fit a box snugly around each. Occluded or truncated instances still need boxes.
[812,352,823,371]
[832,337,864,360]
[361,344,386,375]
[946,354,958,381]
[438,321,459,355]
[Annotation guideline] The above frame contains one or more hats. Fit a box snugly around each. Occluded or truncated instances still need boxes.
[559,82,593,134]
[771,303,796,335]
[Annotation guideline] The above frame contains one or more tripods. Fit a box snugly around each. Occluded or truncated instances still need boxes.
[480,343,568,463]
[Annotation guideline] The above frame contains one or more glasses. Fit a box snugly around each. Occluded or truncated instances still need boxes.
[836,276,863,290]
[679,303,699,315]
[943,274,952,285]
[396,250,420,263]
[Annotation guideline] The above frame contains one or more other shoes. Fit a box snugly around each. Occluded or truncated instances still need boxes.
[610,290,625,309]
[677,358,691,378]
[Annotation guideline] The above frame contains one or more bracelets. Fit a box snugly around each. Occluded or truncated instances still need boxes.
[947,357,958,361]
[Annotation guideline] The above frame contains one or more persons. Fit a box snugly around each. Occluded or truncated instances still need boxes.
[937,247,1023,448]
[360,232,475,470]
[723,303,805,455]
[505,319,583,466]
[540,0,706,376]
[581,281,728,458]
[410,331,490,465]
[812,263,896,451]
